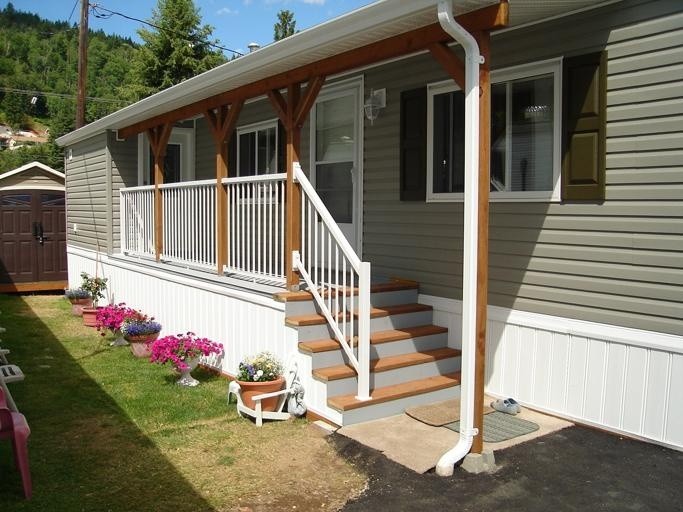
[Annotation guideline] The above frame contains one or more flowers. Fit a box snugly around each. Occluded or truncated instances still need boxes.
[92,301,148,339]
[120,320,163,336]
[147,329,224,369]
[237,350,283,383]
[65,286,92,298]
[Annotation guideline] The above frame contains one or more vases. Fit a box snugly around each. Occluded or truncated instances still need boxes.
[126,335,159,357]
[170,355,200,387]
[70,297,93,316]
[108,324,129,347]
[235,375,286,416]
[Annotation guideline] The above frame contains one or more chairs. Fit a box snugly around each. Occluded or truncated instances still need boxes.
[0,326,35,509]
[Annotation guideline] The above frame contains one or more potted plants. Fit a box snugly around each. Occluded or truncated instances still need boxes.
[79,272,108,326]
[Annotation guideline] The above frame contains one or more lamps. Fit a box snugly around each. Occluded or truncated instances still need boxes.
[361,87,386,125]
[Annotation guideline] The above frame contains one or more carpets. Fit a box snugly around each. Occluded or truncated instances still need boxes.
[405,394,495,429]
[331,394,576,476]
[446,409,538,443]
